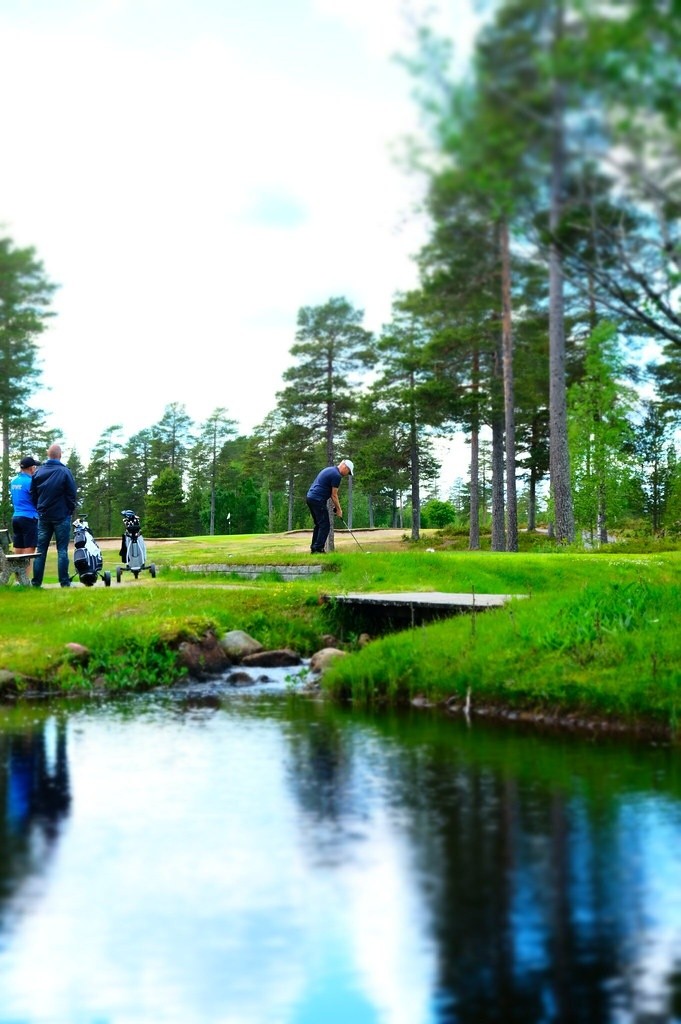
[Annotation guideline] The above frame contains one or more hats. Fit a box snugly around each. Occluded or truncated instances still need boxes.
[19,457,40,469]
[342,459,354,476]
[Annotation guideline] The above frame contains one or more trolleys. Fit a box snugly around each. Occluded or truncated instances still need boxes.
[64,513,111,587]
[117,509,157,583]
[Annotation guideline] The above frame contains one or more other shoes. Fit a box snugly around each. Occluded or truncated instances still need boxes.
[311,549,326,554]
[15,582,19,586]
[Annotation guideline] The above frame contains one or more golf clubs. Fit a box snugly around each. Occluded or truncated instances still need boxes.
[340,517,370,554]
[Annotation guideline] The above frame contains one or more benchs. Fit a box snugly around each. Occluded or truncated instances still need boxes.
[0,547,42,589]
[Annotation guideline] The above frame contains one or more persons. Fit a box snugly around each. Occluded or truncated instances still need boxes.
[11,457,38,587]
[30,444,76,588]
[306,459,354,555]
